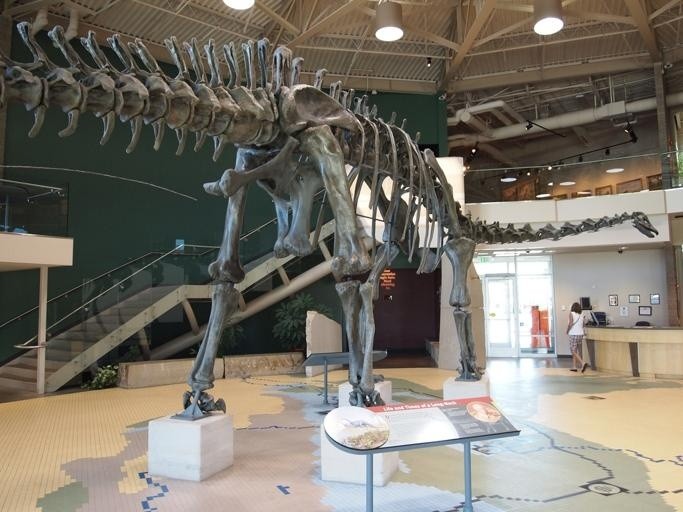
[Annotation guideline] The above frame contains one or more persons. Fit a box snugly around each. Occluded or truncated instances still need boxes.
[564,301,587,375]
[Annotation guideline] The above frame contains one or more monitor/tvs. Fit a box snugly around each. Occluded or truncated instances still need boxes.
[580,297,592,310]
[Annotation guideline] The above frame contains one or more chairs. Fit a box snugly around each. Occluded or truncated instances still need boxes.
[635,321,650,326]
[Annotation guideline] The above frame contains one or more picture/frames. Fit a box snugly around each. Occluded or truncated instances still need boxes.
[609,295,618,306]
[629,294,640,303]
[650,294,660,305]
[638,306,652,316]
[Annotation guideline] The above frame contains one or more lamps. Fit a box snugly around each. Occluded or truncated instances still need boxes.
[375,0,404,42]
[463,118,638,185]
[533,0,564,35]
[426,57,432,67]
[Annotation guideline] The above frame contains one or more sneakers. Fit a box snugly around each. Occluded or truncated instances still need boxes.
[582,362,588,373]
[569,367,579,373]
